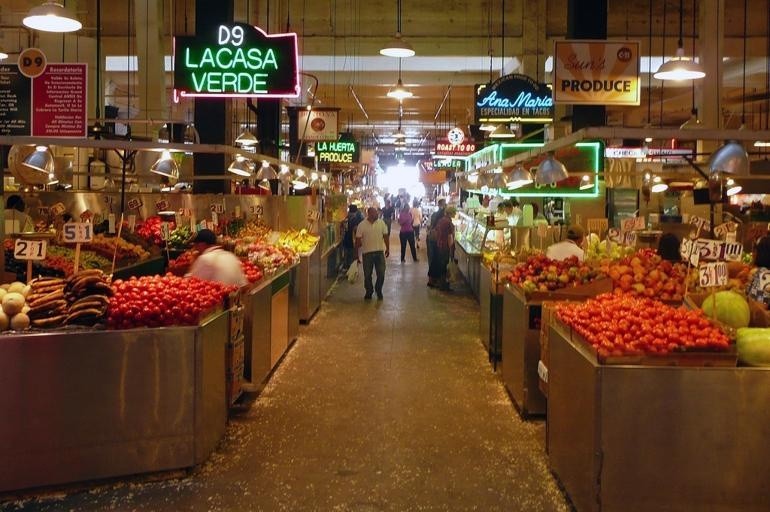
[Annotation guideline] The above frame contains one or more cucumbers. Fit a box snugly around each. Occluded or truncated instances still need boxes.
[27,269,114,329]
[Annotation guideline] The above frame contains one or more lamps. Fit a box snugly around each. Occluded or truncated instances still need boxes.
[384,58,413,158]
[378,0,416,58]
[20,0,84,33]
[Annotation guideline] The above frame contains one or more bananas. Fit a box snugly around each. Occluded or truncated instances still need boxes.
[278,229,319,253]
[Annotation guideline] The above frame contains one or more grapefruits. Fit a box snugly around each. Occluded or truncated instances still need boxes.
[0,282,30,330]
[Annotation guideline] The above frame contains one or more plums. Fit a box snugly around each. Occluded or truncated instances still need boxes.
[506,255,603,291]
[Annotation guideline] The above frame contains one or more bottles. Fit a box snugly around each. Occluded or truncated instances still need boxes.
[522,204,534,226]
[660,215,682,258]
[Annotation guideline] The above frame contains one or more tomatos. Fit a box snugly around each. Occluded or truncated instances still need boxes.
[566,176,579,187]
[106,251,262,328]
[554,293,729,364]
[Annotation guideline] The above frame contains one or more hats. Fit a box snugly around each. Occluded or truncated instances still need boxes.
[190,228,217,244]
[567,225,587,239]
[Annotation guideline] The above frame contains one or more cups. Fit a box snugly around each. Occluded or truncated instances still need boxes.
[166,123,190,142]
[486,216,495,226]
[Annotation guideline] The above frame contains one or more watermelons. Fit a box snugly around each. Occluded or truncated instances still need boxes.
[702,291,749,329]
[736,328,770,364]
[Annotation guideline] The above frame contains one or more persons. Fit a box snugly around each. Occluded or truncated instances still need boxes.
[545,225,586,262]
[4,196,35,235]
[184,229,247,296]
[481,195,546,227]
[346,187,456,299]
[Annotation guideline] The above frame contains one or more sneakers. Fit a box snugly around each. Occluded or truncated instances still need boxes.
[364,289,374,301]
[375,287,383,299]
[428,278,454,292]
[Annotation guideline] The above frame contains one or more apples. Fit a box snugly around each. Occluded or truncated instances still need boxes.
[311,118,325,132]
[599,256,698,300]
